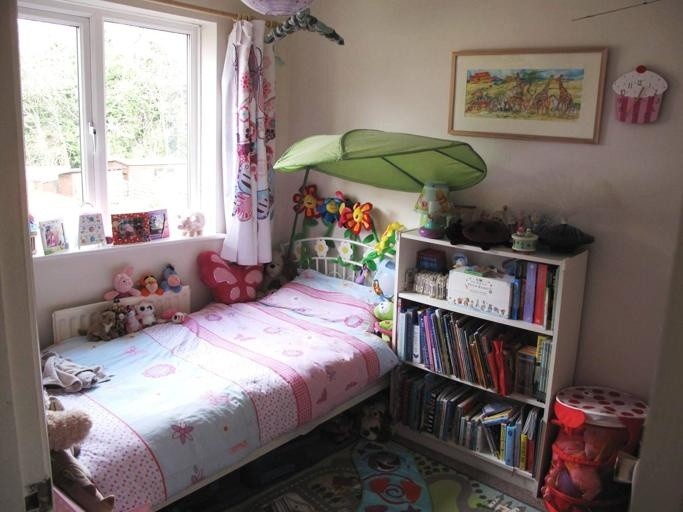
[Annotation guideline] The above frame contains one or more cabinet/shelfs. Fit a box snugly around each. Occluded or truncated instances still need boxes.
[386,225,590,500]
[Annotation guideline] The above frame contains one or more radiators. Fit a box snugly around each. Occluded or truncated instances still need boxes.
[47,285,194,345]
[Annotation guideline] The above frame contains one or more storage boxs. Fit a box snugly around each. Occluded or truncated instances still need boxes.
[449,263,515,319]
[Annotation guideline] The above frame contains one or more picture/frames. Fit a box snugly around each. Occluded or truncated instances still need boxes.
[447,43,609,146]
[39,217,70,258]
[147,209,171,243]
[111,211,151,247]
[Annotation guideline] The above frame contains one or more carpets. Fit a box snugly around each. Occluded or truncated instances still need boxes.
[218,438,545,512]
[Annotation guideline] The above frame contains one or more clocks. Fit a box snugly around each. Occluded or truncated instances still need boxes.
[612,65,668,126]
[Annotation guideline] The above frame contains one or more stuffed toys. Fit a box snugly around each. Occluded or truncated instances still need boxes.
[104,265,142,300]
[102,303,130,338]
[447,219,511,251]
[78,311,115,342]
[135,301,157,328]
[374,256,396,303]
[137,273,164,297]
[44,385,115,511]
[158,265,182,293]
[260,250,283,296]
[157,309,188,323]
[374,302,393,343]
[178,213,205,236]
[125,305,145,333]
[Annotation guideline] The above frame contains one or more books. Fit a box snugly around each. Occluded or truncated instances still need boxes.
[393,365,544,475]
[404,267,448,300]
[396,297,553,404]
[510,259,559,330]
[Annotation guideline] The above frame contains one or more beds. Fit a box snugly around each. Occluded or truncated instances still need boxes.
[39,237,393,512]
